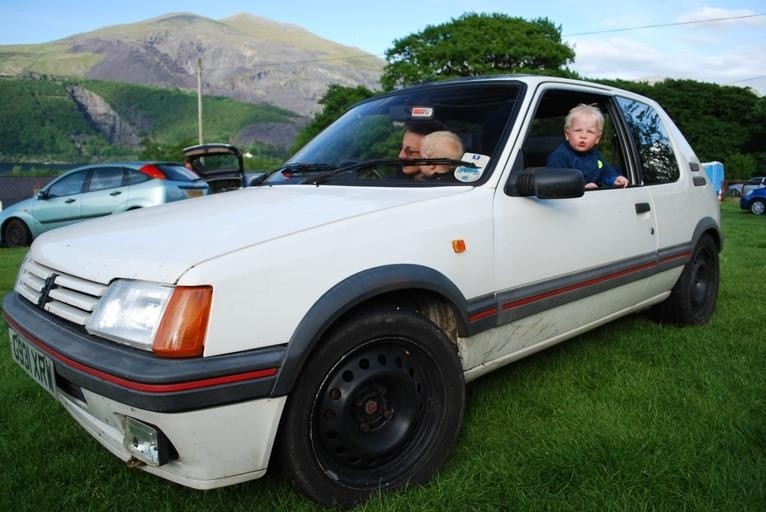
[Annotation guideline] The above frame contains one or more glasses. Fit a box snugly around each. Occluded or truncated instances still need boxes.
[399,143,420,156]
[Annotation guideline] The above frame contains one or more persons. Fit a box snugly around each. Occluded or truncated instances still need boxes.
[543,102,630,190]
[418,130,465,183]
[398,118,452,182]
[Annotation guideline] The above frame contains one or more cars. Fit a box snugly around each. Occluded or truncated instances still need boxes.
[1,161,209,247]
[0,71,727,508]
[739,187,766,215]
[727,176,766,199]
[181,143,265,190]
